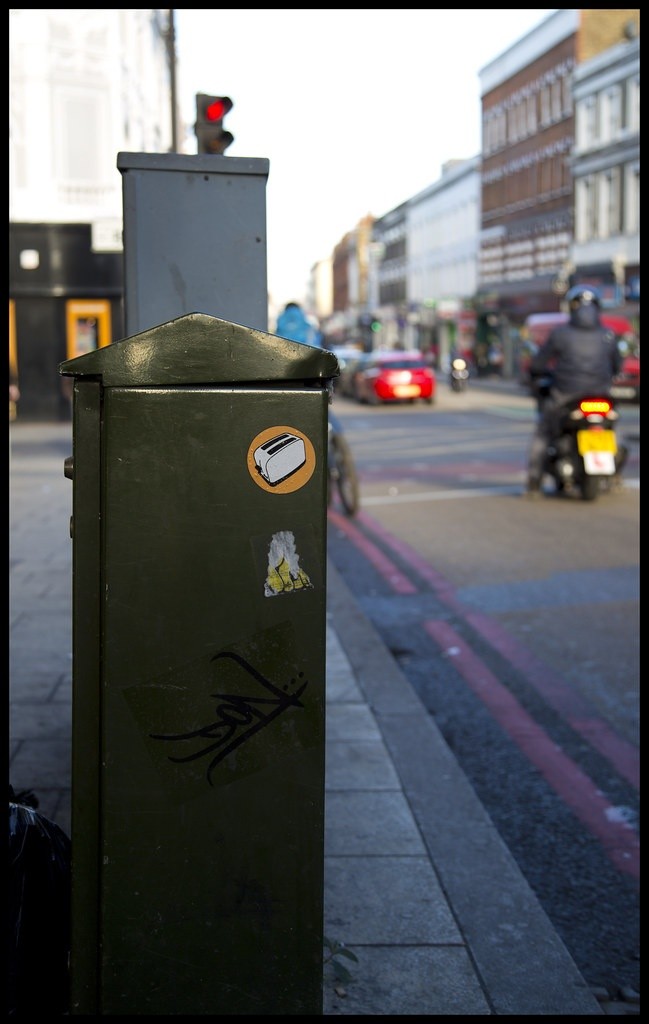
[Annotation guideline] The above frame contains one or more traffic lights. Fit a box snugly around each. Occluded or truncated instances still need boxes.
[195,93,234,156]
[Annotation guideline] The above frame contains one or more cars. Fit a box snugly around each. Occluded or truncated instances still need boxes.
[521,311,642,404]
[351,348,437,407]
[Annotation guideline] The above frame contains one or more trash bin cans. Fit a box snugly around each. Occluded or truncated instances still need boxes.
[52,310,346,1024]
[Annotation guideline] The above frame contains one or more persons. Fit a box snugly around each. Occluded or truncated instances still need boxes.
[527,284,623,492]
[275,303,331,350]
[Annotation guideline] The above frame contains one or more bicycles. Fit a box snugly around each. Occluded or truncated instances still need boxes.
[316,384,361,523]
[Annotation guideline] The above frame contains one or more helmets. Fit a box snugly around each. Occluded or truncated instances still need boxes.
[565,284,602,304]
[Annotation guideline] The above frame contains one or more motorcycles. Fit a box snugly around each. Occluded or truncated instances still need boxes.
[524,339,629,502]
